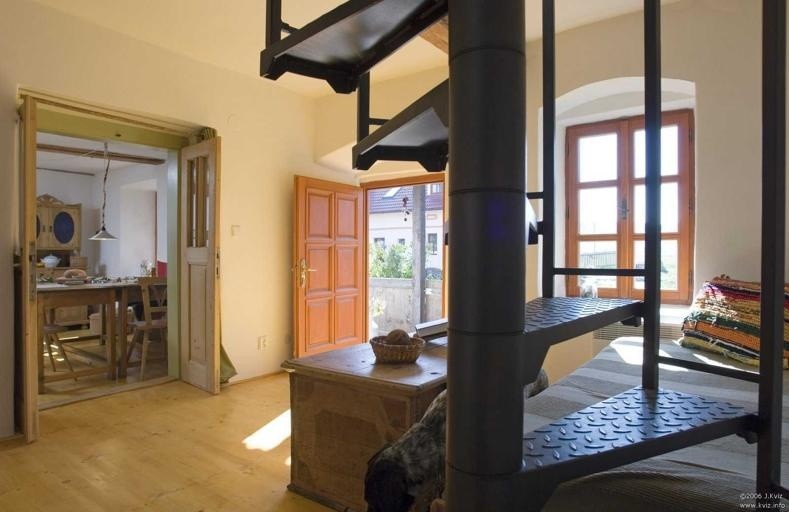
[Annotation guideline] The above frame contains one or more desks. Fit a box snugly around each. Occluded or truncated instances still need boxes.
[38,279,165,395]
[281,336,450,512]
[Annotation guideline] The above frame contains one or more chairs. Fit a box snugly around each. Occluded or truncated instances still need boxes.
[127,276,167,381]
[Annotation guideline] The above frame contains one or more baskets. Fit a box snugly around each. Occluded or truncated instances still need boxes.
[371,335,427,363]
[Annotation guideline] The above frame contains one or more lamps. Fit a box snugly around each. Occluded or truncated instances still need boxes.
[89,153,118,241]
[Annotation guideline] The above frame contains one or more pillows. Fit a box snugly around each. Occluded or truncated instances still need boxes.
[680,274,789,371]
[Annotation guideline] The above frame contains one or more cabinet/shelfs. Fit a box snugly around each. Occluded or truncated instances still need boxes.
[36,192,80,251]
[36,267,89,329]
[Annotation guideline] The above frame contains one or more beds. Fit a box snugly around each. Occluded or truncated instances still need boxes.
[523,334,789,512]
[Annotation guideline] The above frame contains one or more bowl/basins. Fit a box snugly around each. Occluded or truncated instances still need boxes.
[40,259,62,267]
